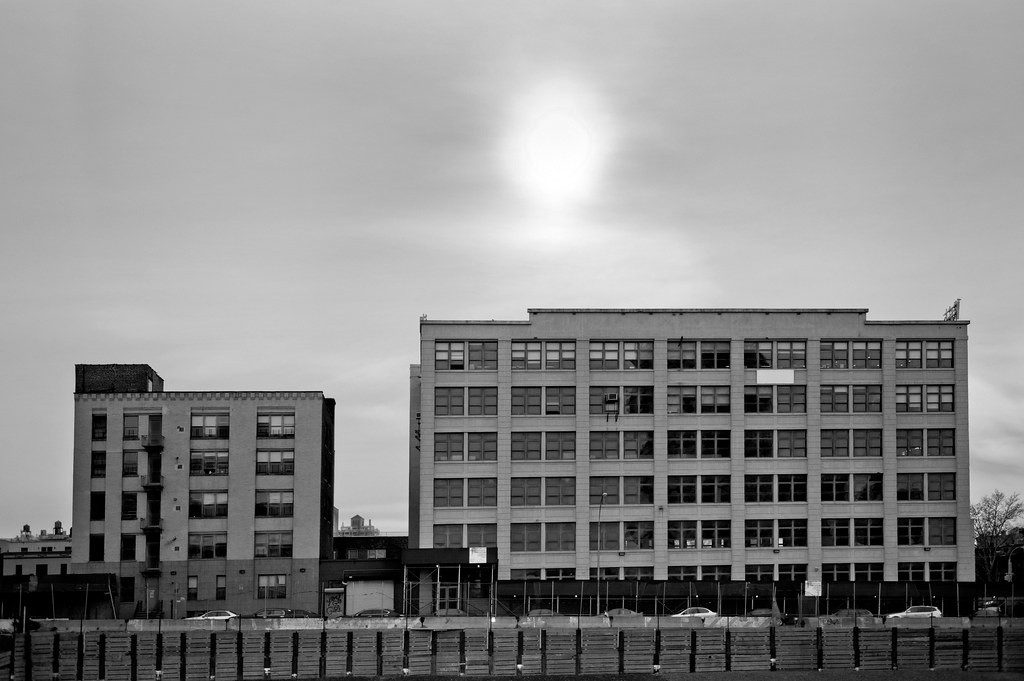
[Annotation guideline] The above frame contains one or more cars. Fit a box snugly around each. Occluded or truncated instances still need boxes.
[670,607,719,617]
[238,607,294,619]
[522,608,564,617]
[597,608,640,617]
[183,609,239,621]
[336,610,403,618]
[737,609,793,626]
[429,608,466,617]
[821,609,875,618]
[292,609,329,621]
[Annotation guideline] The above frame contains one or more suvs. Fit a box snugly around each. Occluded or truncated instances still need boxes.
[886,606,943,619]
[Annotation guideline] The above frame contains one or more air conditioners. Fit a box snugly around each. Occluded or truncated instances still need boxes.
[606,393,617,402]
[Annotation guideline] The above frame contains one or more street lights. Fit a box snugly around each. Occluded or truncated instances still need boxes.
[596,493,609,615]
